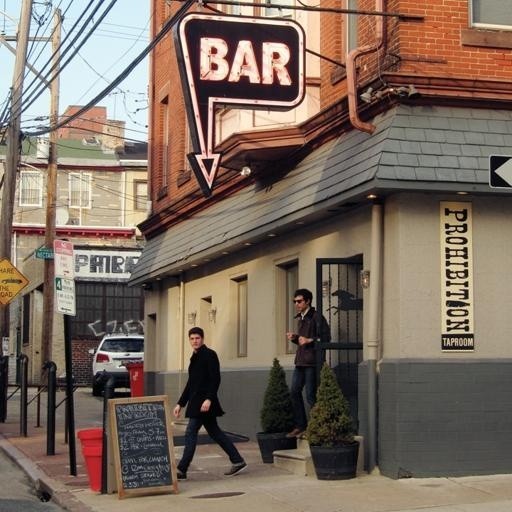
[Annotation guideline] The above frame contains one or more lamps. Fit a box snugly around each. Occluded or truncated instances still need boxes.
[360,87,373,103]
[242,160,251,175]
[208,306,216,321]
[361,270,369,288]
[188,310,196,324]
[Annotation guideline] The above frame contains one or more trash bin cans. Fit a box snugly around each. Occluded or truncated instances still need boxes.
[124,363,144,397]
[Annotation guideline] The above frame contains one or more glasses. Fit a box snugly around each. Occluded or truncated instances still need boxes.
[292,299,304,304]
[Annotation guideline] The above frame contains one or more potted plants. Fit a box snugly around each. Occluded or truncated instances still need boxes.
[256,357,297,463]
[306,362,359,480]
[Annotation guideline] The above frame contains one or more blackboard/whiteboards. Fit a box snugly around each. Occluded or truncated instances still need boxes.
[107,395,177,495]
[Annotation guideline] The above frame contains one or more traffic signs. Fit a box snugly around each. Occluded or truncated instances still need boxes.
[0,257,28,305]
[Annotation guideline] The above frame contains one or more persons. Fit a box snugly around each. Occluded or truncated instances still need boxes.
[286,289,331,439]
[173,326,248,481]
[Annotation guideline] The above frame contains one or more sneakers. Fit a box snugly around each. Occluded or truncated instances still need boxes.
[286,427,307,439]
[223,461,248,477]
[176,472,187,480]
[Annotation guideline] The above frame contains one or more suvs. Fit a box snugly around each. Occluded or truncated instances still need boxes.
[86,334,145,395]
